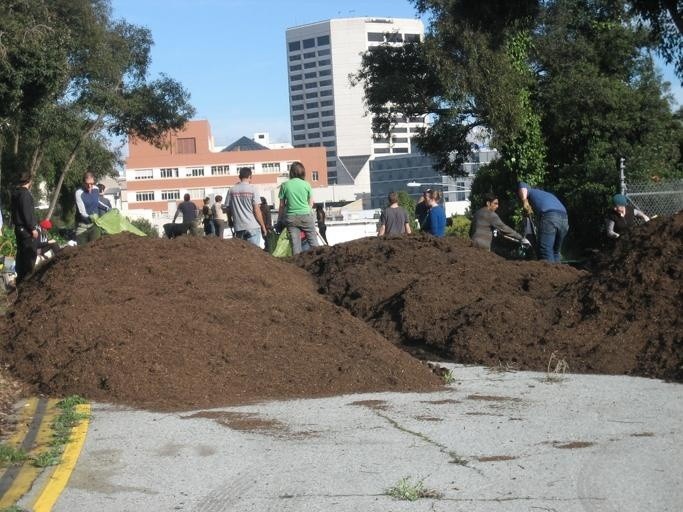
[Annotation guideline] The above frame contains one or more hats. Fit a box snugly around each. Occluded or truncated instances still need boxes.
[611,195,627,206]
[38,221,51,229]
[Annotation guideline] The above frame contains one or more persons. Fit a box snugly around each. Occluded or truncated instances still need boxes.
[378,192,411,238]
[172,161,318,256]
[518,178,569,264]
[424,190,447,238]
[604,194,649,242]
[0,169,113,287]
[316,204,328,246]
[469,194,531,252]
[416,189,432,230]
